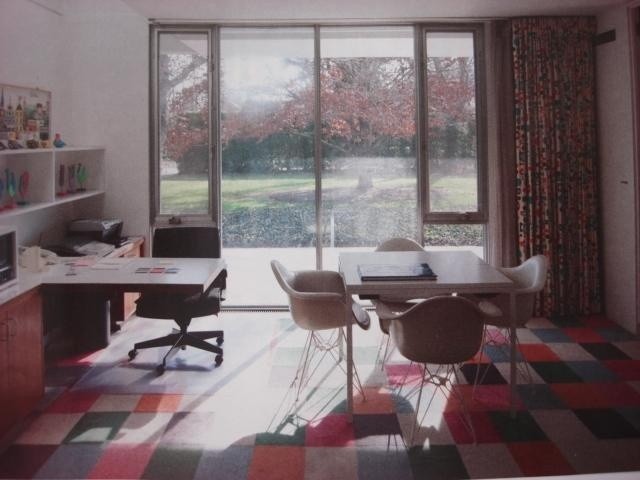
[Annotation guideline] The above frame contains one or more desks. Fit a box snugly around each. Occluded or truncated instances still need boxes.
[1,241,227,284]
[333,248,517,422]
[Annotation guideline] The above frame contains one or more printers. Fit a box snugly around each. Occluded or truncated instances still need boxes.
[67,216,124,248]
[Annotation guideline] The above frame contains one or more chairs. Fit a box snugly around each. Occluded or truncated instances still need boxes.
[271,258,372,403]
[126,227,229,375]
[458,254,549,400]
[365,237,430,372]
[374,293,504,451]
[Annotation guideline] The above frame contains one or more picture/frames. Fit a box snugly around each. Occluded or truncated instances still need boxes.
[0,82,55,147]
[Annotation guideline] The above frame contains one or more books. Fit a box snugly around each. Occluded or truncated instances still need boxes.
[358,262,438,281]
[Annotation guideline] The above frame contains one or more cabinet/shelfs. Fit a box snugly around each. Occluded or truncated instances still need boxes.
[111,236,143,321]
[1,146,107,217]
[0,285,44,453]
